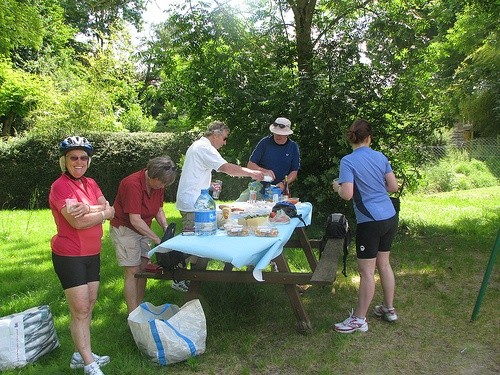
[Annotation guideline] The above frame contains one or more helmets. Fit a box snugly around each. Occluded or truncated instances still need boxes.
[59,136,92,152]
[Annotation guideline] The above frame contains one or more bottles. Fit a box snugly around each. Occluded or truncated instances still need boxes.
[194,189,216,236]
[283,176,289,202]
[249,179,257,200]
[264,186,283,202]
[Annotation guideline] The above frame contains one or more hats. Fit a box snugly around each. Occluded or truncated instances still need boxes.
[269,118,292,135]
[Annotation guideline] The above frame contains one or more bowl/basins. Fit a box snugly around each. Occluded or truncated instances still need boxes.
[263,176,272,181]
[288,198,299,205]
[247,216,268,227]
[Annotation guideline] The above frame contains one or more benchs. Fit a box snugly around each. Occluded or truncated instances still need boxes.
[135,269,157,279]
[309,236,343,286]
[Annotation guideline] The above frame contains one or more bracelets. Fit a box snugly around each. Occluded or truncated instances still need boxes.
[101,211,105,219]
[86,204,90,214]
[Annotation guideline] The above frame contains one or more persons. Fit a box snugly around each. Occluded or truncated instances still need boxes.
[333,119,399,334]
[110,155,178,312]
[236,117,300,202]
[49,136,115,375]
[171,120,264,293]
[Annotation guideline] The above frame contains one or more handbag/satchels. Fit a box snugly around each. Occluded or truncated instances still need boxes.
[154,223,186,270]
[129,299,208,365]
[0,304,61,370]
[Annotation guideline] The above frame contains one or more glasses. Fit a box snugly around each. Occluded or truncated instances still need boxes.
[219,135,227,142]
[69,156,89,162]
[274,122,289,128]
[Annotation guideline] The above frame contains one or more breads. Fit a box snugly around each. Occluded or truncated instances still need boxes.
[228,229,249,237]
[255,227,278,237]
[219,205,246,211]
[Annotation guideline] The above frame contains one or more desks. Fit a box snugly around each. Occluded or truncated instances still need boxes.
[147,202,325,338]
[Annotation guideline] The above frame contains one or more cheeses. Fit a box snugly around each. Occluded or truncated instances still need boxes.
[247,216,269,227]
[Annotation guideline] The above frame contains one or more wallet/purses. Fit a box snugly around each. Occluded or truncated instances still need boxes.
[146,263,164,273]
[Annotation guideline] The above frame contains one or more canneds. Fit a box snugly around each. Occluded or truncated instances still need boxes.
[250,190,257,203]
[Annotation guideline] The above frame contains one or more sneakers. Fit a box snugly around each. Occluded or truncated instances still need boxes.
[333,309,369,334]
[70,350,110,370]
[83,362,104,375]
[373,305,397,321]
[172,280,188,291]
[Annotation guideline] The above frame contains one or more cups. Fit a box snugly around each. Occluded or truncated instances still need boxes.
[66,199,77,213]
[334,178,341,192]
[213,185,222,199]
[273,194,278,203]
